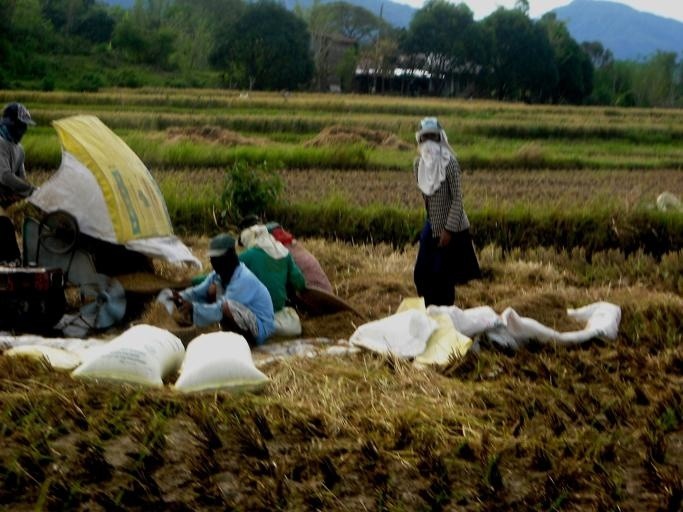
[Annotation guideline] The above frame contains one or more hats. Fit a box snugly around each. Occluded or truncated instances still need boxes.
[416,115,442,139]
[204,232,237,258]
[3,101,39,126]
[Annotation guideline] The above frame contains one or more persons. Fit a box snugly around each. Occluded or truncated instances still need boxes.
[180,214,307,315]
[263,220,335,296]
[0,101,42,266]
[166,233,276,354]
[411,116,480,307]
